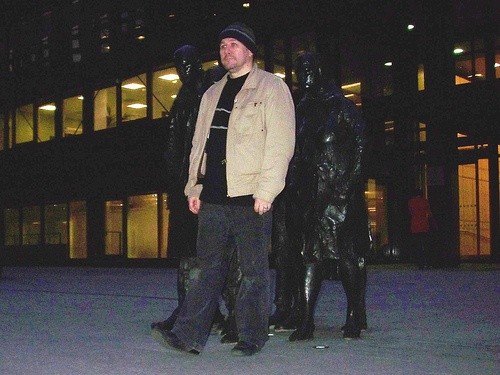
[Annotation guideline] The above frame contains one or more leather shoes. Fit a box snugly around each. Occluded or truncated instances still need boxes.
[231,341,261,356]
[151,324,200,357]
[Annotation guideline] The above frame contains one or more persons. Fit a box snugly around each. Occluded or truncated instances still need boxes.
[151,44,226,337]
[405,188,439,270]
[149,22,299,357]
[287,51,374,342]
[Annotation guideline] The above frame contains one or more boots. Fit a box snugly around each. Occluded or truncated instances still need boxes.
[151,255,197,327]
[340,254,368,340]
[289,260,325,342]
[268,263,298,331]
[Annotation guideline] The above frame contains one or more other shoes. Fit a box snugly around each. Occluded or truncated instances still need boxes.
[219,318,237,344]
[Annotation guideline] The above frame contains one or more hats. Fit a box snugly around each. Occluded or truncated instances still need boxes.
[217,22,256,54]
